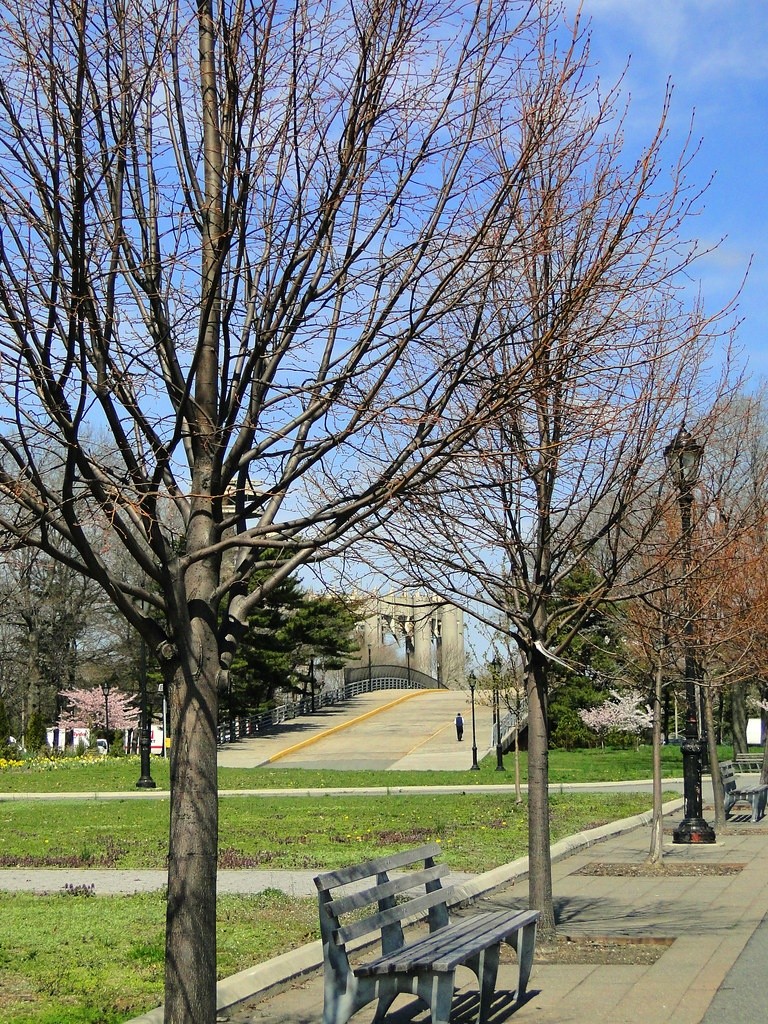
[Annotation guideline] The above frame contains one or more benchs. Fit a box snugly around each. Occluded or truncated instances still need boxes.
[313,841,543,1024]
[731,752,764,773]
[719,760,768,823]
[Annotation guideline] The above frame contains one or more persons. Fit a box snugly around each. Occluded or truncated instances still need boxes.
[454,713,464,741]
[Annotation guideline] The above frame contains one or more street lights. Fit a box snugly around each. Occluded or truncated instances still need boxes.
[661,418,719,845]
[488,654,507,770]
[406,647,412,689]
[100,678,112,754]
[468,669,481,770]
[436,662,441,689]
[309,647,317,713]
[367,642,372,692]
